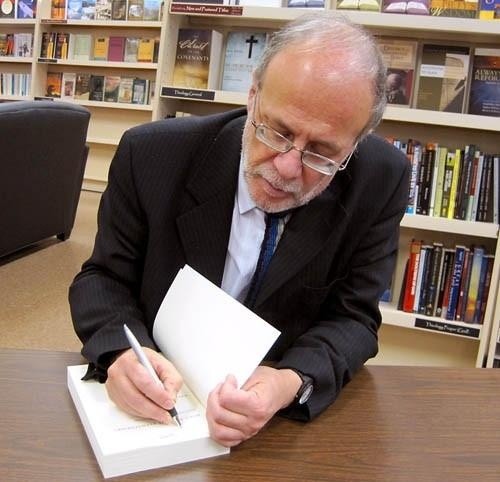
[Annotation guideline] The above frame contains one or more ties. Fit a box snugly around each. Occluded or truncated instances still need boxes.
[244,212,290,309]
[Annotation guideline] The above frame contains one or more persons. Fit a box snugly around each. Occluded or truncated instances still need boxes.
[67,8,415,448]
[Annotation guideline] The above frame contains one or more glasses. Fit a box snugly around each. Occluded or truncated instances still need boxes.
[251,87,360,177]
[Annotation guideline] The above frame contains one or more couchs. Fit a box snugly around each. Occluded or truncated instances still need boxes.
[0,100,90,255]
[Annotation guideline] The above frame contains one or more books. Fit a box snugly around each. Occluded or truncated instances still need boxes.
[0,0,162,106]
[375,37,500,326]
[172,0,327,8]
[64,264,283,479]
[173,30,271,94]
[336,0,500,19]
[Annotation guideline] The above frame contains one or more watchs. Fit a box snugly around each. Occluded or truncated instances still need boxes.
[291,368,314,405]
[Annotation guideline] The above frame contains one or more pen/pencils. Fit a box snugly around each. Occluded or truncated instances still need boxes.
[121,323,183,428]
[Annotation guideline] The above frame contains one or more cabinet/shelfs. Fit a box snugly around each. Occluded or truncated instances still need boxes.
[151,1,500,368]
[0,2,168,194]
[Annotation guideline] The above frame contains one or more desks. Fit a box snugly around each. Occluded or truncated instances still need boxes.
[0,348,500,482]
